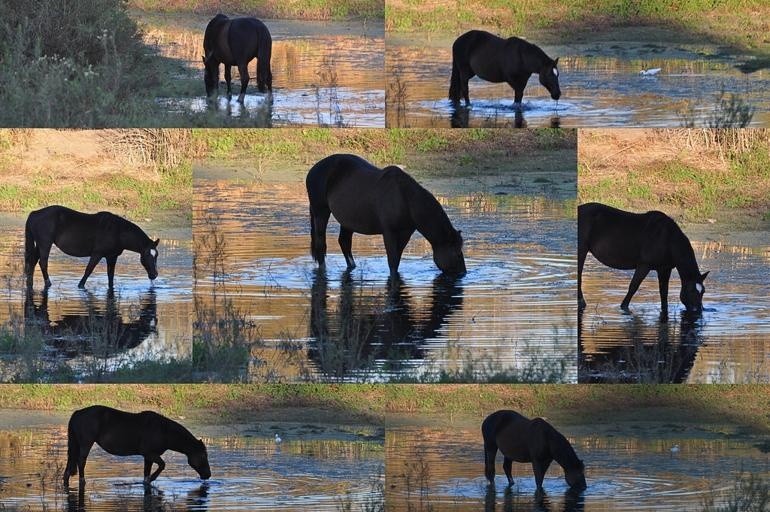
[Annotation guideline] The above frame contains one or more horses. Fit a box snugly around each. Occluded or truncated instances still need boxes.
[447,28,561,109]
[61,405,212,489]
[201,14,274,105]
[24,204,159,291]
[305,153,467,280]
[578,202,711,314]
[479,408,588,493]
